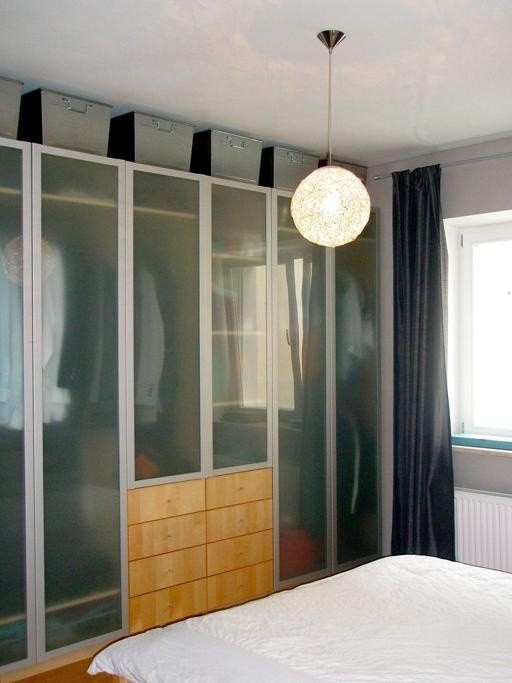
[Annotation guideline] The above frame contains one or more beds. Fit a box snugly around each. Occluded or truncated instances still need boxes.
[85,552,512,683]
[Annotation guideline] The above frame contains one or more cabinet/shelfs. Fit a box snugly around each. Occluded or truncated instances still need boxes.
[1,136,383,683]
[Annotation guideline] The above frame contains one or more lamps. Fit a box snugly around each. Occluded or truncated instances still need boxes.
[288,27,372,250]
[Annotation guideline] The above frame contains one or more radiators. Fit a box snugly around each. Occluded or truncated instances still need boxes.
[453,488,512,573]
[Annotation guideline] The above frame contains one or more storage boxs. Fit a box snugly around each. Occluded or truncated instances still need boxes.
[2,72,264,185]
